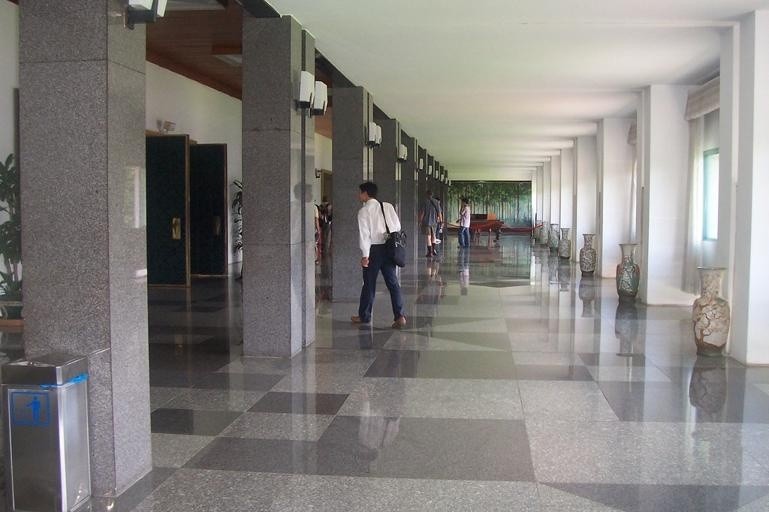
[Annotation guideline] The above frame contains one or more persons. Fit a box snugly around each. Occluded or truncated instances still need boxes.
[357,324,406,461]
[419,191,442,257]
[457,248,471,295]
[318,196,332,257]
[435,198,444,255]
[291,183,316,315]
[318,254,333,303]
[351,182,407,329]
[427,257,443,305]
[457,198,470,248]
[313,204,320,264]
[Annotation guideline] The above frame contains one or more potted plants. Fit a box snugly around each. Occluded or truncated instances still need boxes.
[0,153,28,327]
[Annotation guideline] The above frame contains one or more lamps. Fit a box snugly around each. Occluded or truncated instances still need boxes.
[365,121,383,149]
[294,69,328,118]
[418,158,452,187]
[397,143,408,163]
[123,1,170,30]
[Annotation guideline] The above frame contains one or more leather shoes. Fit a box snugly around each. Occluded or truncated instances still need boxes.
[425,249,437,256]
[350,315,362,322]
[392,316,406,328]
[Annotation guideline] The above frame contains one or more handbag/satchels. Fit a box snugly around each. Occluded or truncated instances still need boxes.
[390,231,407,267]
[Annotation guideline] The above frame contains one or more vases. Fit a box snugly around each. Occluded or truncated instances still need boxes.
[533,220,572,260]
[691,266,731,356]
[615,243,641,302]
[579,234,597,276]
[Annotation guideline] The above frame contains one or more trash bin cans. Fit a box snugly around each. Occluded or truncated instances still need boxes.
[1,353,92,512]
